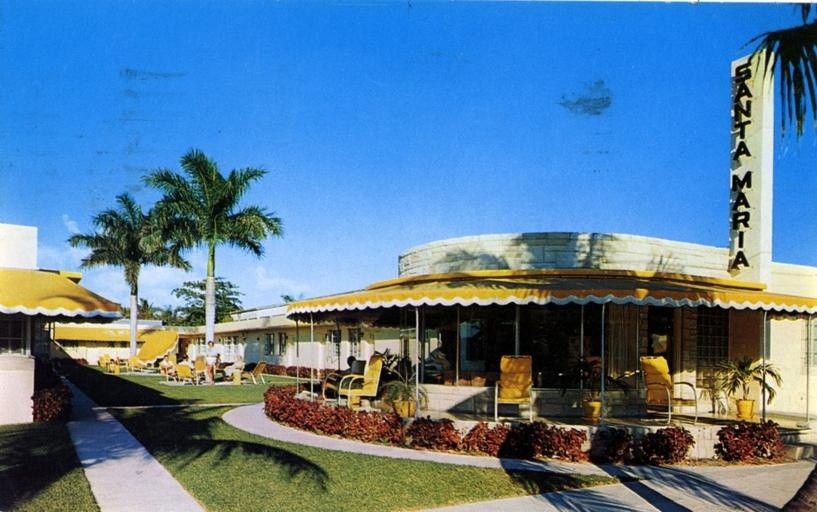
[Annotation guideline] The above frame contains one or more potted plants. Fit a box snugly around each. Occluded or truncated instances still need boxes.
[378,366,427,416]
[555,356,631,423]
[718,356,783,418]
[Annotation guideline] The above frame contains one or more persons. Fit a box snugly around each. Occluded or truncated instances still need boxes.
[223,356,246,380]
[178,354,195,370]
[162,355,176,376]
[206,341,216,353]
[336,356,356,381]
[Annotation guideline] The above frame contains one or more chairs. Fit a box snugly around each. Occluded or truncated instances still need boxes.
[492,354,534,422]
[320,360,364,402]
[336,354,383,409]
[159,354,267,387]
[637,333,698,425]
[96,354,142,373]
